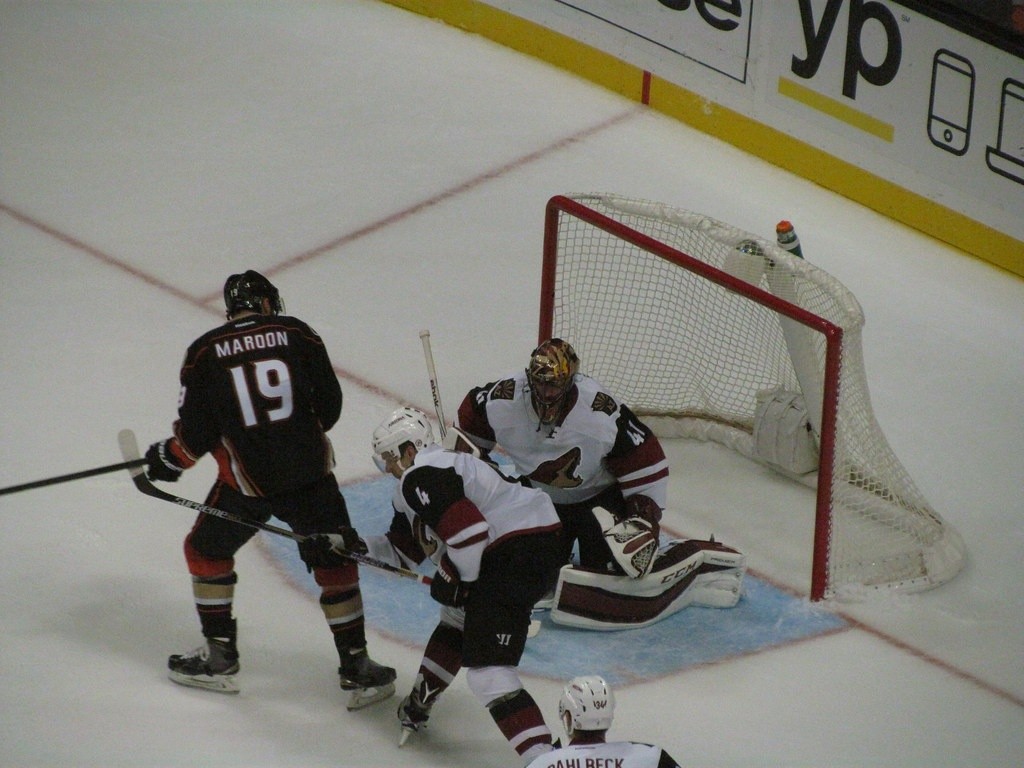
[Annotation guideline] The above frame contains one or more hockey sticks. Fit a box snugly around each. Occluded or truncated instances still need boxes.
[119,429,433,584]
[0,457,144,497]
[418,329,446,439]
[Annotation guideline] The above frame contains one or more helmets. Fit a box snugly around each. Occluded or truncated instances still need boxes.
[223,269,281,319]
[372,407,434,466]
[524,338,579,424]
[559,674,615,737]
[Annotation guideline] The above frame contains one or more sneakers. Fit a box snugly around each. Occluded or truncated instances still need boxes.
[336,642,397,712]
[168,618,240,692]
[397,672,437,747]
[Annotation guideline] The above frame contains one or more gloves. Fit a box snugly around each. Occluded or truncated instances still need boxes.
[297,527,368,574]
[144,437,186,482]
[430,552,476,609]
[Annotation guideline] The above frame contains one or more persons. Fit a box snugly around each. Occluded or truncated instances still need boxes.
[525,675,682,768]
[146,270,398,709]
[458,338,745,631]
[300,405,561,765]
